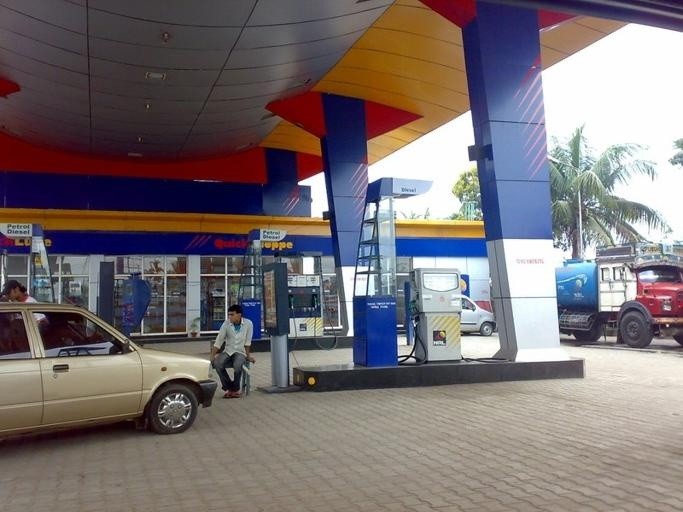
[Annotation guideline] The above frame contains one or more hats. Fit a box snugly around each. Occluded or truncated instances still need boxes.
[1,279,20,295]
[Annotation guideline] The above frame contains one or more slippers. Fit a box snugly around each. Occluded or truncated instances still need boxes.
[222,393,240,399]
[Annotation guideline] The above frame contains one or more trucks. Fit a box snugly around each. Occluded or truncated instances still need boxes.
[554,239,682,349]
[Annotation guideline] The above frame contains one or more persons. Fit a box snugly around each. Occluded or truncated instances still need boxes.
[209,303,256,399]
[0,278,50,328]
[43,315,97,351]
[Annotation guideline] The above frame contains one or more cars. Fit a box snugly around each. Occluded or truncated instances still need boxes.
[0,301,219,442]
[461,294,498,336]
